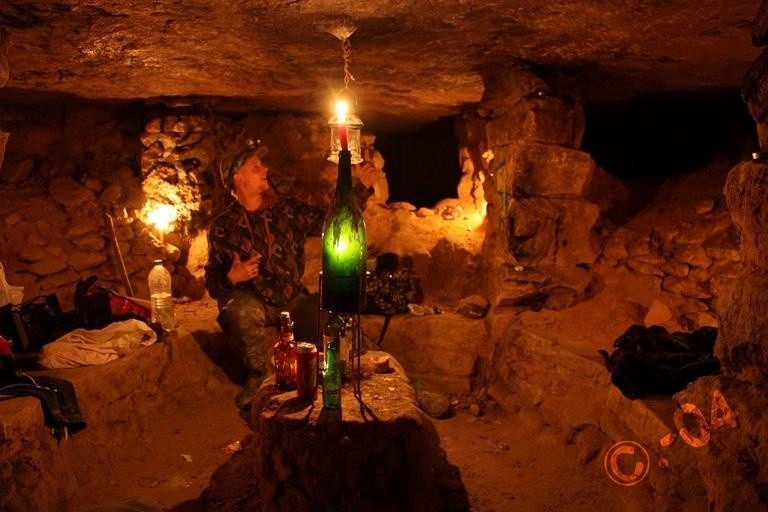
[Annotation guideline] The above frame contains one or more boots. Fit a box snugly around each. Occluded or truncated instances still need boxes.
[235,377,262,410]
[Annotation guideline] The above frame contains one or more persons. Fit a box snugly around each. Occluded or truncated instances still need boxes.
[197,140,321,391]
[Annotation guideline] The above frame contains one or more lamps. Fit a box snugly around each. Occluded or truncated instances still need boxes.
[327,25,363,164]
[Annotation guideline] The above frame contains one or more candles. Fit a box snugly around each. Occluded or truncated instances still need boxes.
[336,100,349,152]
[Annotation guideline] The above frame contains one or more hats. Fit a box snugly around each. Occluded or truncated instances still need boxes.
[219,146,269,188]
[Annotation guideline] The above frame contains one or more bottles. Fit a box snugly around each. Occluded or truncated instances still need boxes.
[272,310,295,390]
[323,342,340,409]
[323,310,345,376]
[321,149,371,316]
[148,259,178,333]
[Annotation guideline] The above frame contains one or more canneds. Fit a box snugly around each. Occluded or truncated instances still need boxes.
[296,342,319,401]
